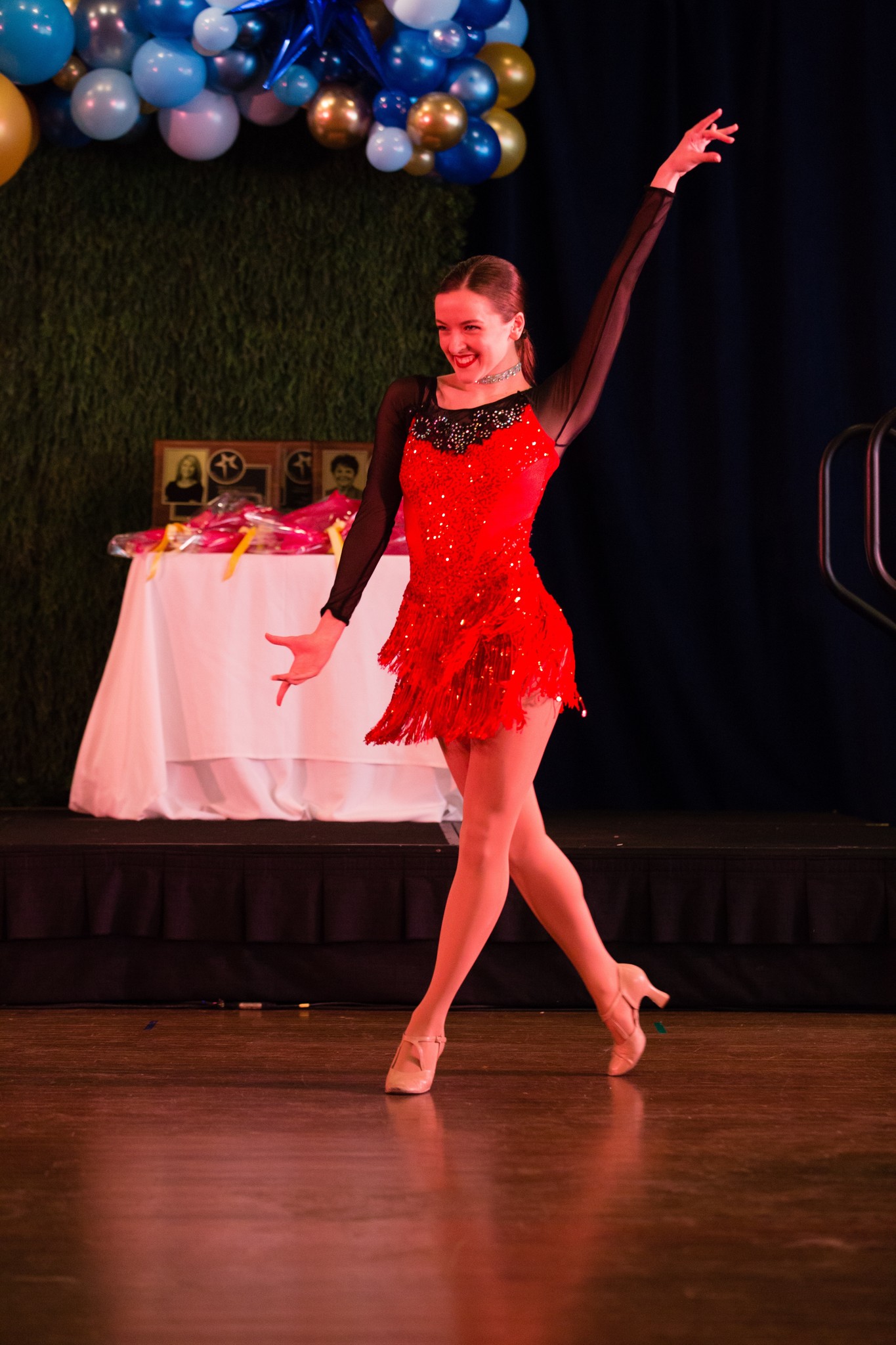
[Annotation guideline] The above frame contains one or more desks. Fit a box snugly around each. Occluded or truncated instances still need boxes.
[66,550,468,824]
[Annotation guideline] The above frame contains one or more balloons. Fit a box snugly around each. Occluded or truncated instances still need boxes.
[0,0,538,183]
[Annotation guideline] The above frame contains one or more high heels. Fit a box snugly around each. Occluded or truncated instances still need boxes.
[383,1030,447,1094]
[599,963,669,1077]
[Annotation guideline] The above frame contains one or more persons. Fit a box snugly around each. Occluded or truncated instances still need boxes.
[165,455,204,517]
[262,109,742,1095]
[325,454,364,499]
